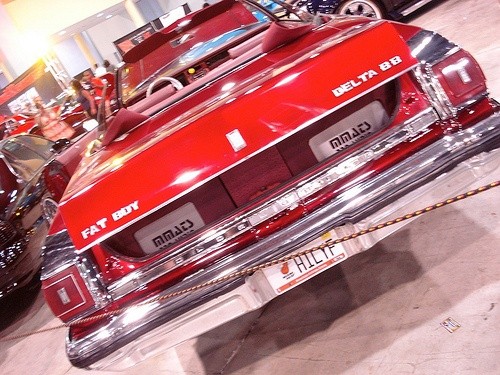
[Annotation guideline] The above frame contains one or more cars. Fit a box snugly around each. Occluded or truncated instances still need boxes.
[282,0,449,25]
[0,131,74,303]
[0,72,116,142]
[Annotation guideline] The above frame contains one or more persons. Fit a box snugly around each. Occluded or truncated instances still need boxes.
[70,60,117,120]
[34,95,75,141]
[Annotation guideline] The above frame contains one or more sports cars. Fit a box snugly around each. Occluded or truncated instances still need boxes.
[39,1,500,373]
